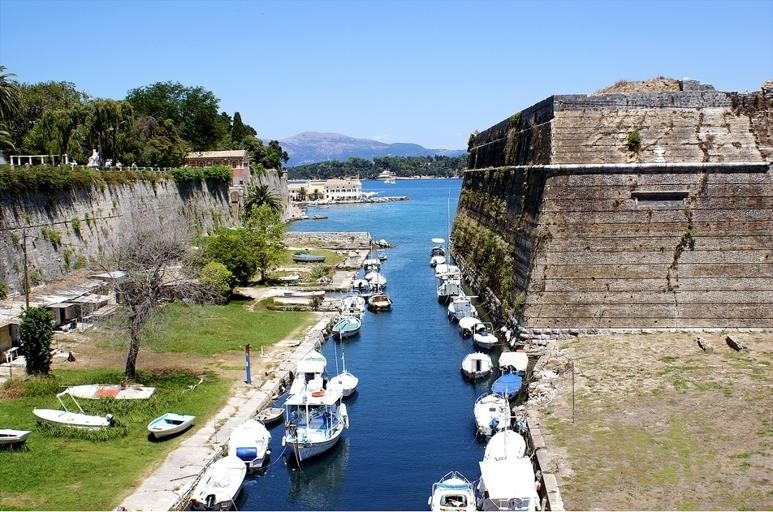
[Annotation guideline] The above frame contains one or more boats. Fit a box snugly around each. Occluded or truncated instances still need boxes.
[253,406,285,424]
[226,419,271,475]
[0,429,31,446]
[65,384,155,401]
[146,412,196,439]
[188,455,247,510]
[32,388,113,431]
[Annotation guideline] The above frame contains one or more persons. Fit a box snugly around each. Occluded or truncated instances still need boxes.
[105,159,112,169]
[131,162,138,171]
[115,159,122,171]
[89,149,98,161]
